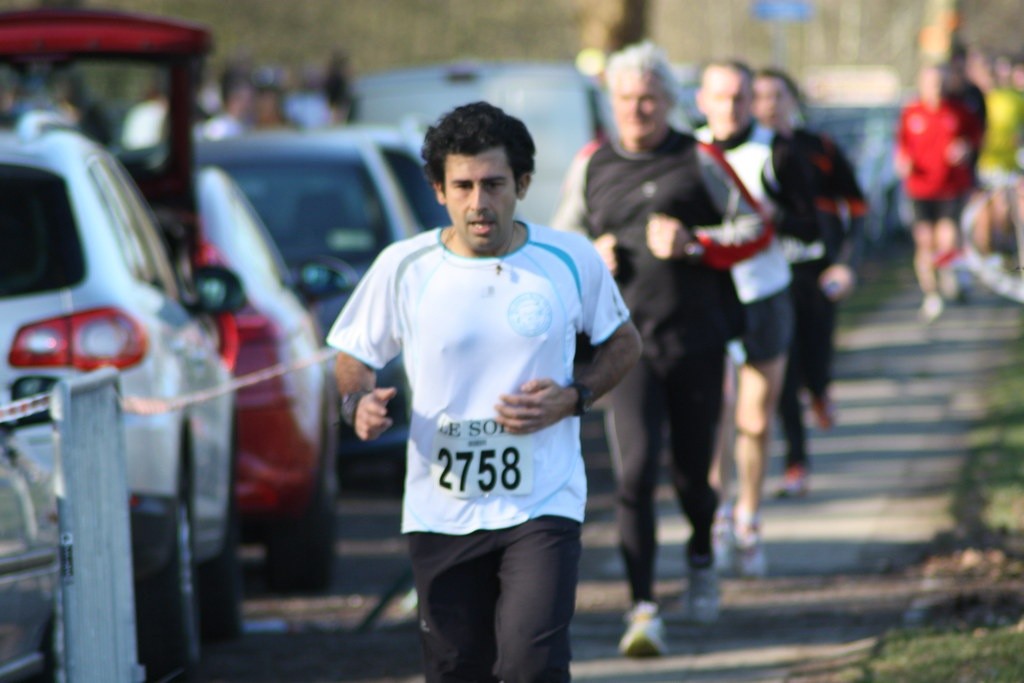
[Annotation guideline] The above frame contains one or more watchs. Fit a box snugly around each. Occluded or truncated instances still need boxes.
[567,383,593,416]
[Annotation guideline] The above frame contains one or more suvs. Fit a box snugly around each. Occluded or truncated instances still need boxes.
[1,109,252,683]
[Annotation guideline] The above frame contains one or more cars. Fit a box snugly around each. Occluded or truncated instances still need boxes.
[1,417,67,683]
[115,129,451,496]
[337,59,913,356]
[0,3,349,596]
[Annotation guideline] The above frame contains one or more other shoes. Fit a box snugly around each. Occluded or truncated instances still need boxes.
[735,537,768,580]
[780,465,808,499]
[812,396,833,433]
[713,528,735,573]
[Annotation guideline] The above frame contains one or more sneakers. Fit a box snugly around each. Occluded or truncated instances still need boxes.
[673,568,720,626]
[618,601,669,655]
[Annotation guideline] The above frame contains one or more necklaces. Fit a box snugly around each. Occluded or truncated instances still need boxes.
[441,221,515,274]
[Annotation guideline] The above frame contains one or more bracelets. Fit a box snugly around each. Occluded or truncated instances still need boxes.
[340,389,372,426]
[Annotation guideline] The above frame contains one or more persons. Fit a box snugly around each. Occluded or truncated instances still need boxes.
[549,38,775,656]
[325,102,645,683]
[687,56,870,577]
[892,49,1024,324]
[204,66,303,140]
[1,74,114,144]
